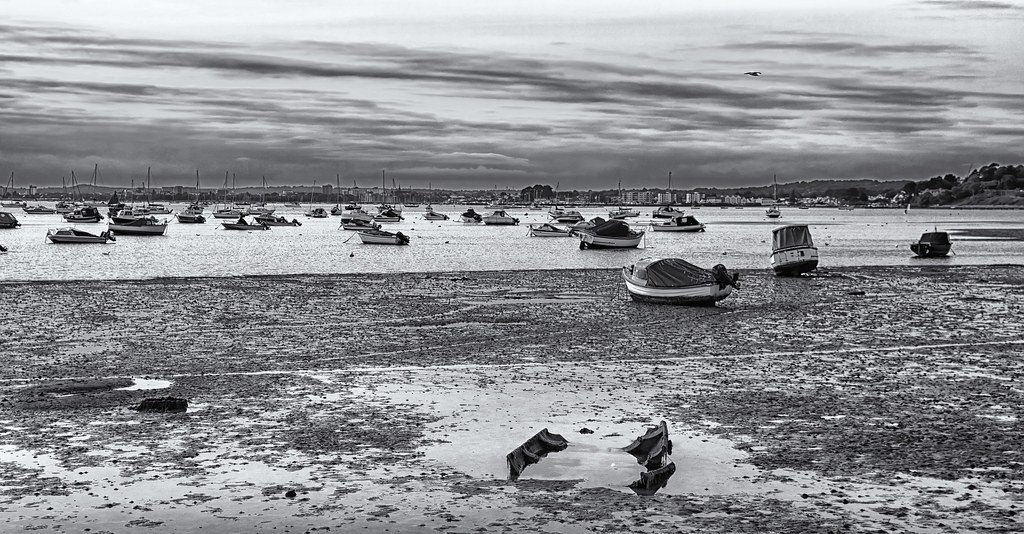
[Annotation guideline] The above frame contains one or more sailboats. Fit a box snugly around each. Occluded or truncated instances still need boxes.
[0,162,420,245]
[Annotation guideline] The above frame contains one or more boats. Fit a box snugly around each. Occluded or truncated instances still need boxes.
[765,202,781,217]
[622,255,741,303]
[505,421,676,497]
[422,208,450,220]
[528,179,707,248]
[460,207,482,223]
[483,207,521,225]
[771,223,819,274]
[910,232,951,255]
